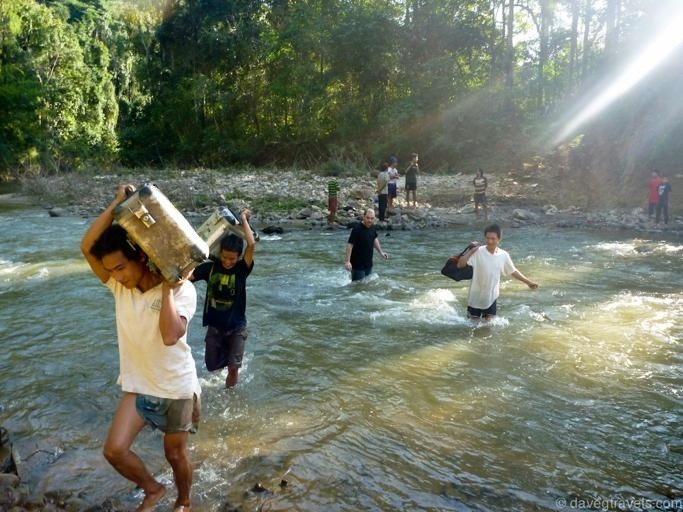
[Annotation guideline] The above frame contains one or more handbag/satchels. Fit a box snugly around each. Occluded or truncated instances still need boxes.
[441,241,477,282]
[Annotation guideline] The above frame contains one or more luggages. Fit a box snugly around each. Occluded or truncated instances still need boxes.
[111,183,259,287]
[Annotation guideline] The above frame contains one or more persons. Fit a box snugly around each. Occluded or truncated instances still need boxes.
[345,208,388,281]
[188,208,255,388]
[386,156,400,215]
[328,172,340,225]
[377,160,390,221]
[647,170,671,224]
[457,224,538,320]
[80,184,201,512]
[473,167,487,208]
[404,152,420,209]
